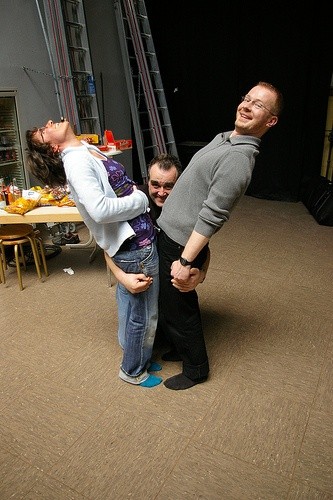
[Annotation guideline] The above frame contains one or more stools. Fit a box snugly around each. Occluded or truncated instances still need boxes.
[0,224,49,291]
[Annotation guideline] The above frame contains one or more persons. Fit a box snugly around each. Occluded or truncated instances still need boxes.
[104,153,210,292]
[156,82,294,391]
[25,119,163,388]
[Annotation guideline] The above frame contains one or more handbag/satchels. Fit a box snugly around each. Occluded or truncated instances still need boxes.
[302,172,333,227]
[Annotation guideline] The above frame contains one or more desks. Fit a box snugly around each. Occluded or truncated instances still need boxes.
[101,150,123,158]
[0,206,112,287]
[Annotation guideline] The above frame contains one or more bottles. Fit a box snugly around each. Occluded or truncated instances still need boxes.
[13,177,21,200]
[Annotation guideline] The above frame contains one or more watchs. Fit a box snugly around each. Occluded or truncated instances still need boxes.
[180,257,192,266]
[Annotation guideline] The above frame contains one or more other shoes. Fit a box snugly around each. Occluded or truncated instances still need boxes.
[59,222,77,233]
[52,232,81,246]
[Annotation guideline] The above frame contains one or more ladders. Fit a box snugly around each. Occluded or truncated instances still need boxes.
[42,0,104,149]
[112,0,181,189]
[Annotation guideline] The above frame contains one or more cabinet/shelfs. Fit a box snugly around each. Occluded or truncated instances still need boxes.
[0,87,30,190]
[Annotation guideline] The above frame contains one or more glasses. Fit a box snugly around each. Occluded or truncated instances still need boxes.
[39,126,47,143]
[241,96,276,116]
